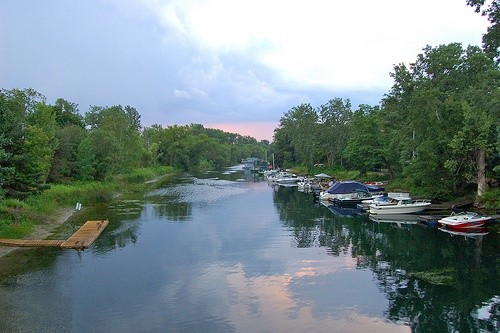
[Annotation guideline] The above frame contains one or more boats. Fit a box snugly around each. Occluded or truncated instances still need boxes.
[239,150,433,217]
[436,210,492,230]
